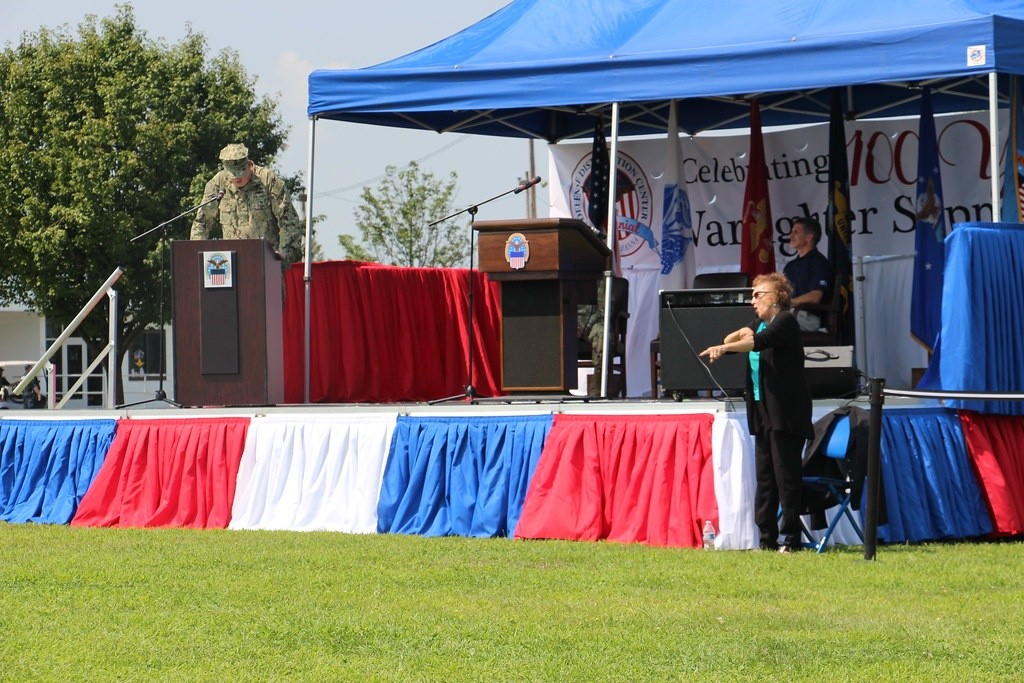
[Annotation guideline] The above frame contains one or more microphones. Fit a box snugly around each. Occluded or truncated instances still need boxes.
[217,188,226,202]
[515,176,541,194]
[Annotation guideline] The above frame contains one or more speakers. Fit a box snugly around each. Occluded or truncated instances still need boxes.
[660,287,760,391]
[803,345,859,399]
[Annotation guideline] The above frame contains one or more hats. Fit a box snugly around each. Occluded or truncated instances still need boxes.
[218,143,250,177]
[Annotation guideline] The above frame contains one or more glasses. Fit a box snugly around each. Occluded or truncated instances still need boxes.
[751,291,775,300]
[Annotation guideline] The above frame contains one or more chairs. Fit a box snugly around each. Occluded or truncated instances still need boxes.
[777,406,869,554]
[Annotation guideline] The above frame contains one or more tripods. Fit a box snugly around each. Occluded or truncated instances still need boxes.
[114,193,218,410]
[428,190,517,405]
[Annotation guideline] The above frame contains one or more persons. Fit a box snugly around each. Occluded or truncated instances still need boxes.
[191,143,303,313]
[784,217,827,331]
[0,364,41,409]
[697,274,814,553]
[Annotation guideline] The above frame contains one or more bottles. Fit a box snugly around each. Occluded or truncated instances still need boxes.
[703,521,716,550]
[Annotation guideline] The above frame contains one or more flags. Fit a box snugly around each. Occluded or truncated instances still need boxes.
[660,100,698,289]
[741,100,776,288]
[588,113,624,277]
[911,88,947,394]
[827,89,855,346]
